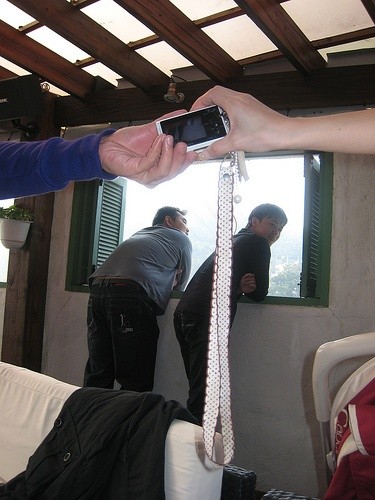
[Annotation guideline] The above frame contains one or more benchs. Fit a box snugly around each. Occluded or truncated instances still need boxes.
[0,361,224,500]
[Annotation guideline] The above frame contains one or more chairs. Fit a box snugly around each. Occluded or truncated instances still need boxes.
[312,332,375,495]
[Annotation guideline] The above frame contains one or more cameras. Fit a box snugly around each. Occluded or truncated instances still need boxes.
[155,104,231,152]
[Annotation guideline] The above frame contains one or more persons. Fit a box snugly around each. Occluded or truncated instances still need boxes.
[0,110,197,201]
[83,206,192,393]
[189,85,374,161]
[172,203,288,427]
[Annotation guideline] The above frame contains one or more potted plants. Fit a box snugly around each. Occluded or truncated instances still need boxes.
[0,204,33,250]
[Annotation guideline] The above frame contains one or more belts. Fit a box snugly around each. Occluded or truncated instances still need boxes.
[91,278,150,300]
